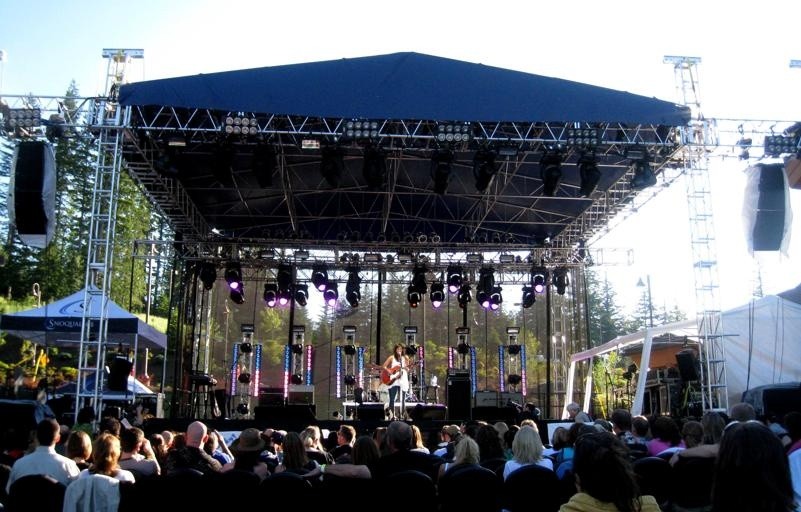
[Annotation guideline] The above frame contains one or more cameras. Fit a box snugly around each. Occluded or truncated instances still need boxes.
[511,400,523,411]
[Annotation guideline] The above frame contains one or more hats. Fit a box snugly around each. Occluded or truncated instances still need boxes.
[442,425,461,437]
[231,428,270,452]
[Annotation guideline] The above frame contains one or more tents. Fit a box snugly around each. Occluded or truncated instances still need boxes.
[0,284,167,403]
[561,282,801,417]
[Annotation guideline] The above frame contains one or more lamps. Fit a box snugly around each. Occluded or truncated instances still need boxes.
[345,121,379,139]
[8,109,41,127]
[225,117,258,135]
[567,129,600,146]
[765,136,797,153]
[437,124,471,142]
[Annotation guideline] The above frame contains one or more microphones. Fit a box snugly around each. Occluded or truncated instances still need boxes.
[398,352,402,363]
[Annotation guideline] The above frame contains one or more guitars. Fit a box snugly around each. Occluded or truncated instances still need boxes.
[382,359,423,386]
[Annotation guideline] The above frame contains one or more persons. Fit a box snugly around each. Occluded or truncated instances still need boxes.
[382,342,410,421]
[0,364,801,511]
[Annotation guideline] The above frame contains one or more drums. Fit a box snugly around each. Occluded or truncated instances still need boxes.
[367,378,398,402]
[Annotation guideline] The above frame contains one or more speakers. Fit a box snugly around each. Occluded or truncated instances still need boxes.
[752,163,786,251]
[447,377,472,421]
[254,405,316,432]
[14,140,48,235]
[675,349,699,383]
[108,358,133,392]
[357,404,385,423]
